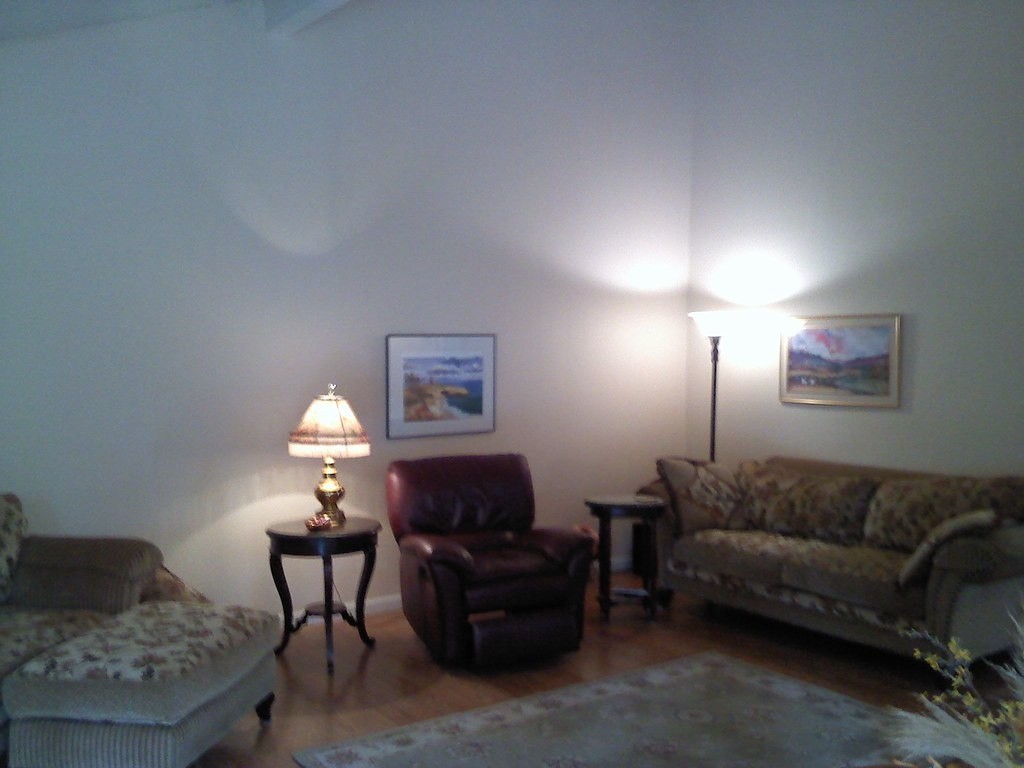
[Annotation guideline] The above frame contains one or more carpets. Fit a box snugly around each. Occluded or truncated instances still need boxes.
[292,645,935,767]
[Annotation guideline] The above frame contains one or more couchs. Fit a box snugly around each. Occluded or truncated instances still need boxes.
[0,491,167,748]
[385,454,599,674]
[638,455,1024,690]
[2,596,285,768]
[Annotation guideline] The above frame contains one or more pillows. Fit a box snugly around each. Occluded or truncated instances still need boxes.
[898,507,999,589]
[657,457,748,535]
[862,474,1024,552]
[737,458,883,545]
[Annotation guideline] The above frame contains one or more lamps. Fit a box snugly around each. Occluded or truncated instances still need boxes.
[688,309,741,463]
[287,381,372,528]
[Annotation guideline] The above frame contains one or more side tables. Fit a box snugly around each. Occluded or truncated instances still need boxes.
[584,493,669,625]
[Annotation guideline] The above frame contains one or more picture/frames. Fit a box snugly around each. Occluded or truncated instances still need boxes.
[385,333,497,439]
[779,313,901,409]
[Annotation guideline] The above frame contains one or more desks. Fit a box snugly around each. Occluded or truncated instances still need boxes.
[265,514,384,677]
